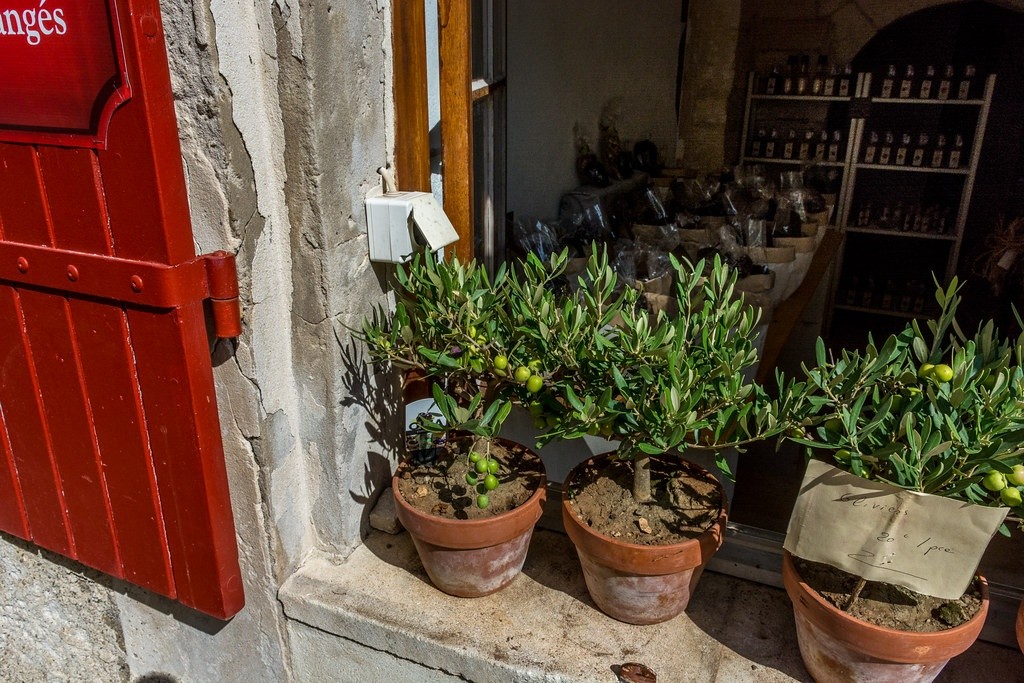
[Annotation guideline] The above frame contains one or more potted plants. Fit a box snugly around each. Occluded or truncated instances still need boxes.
[355,243,577,596]
[543,249,802,625]
[778,273,1024,683]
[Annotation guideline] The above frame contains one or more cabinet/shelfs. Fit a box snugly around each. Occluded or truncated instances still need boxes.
[735,70,998,321]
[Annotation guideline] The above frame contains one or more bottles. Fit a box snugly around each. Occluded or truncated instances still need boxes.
[939,65,955,100]
[879,130,894,165]
[912,133,930,167]
[919,65,934,99]
[824,54,839,97]
[958,67,975,100]
[827,129,841,162]
[781,59,795,96]
[839,64,854,97]
[899,65,915,98]
[814,128,828,161]
[752,128,765,158]
[948,134,964,169]
[864,130,880,164]
[799,130,814,161]
[894,132,912,166]
[795,57,809,95]
[855,200,949,235]
[764,128,779,158]
[764,64,780,96]
[881,65,896,98]
[931,136,947,168]
[810,55,826,96]
[783,130,797,160]
